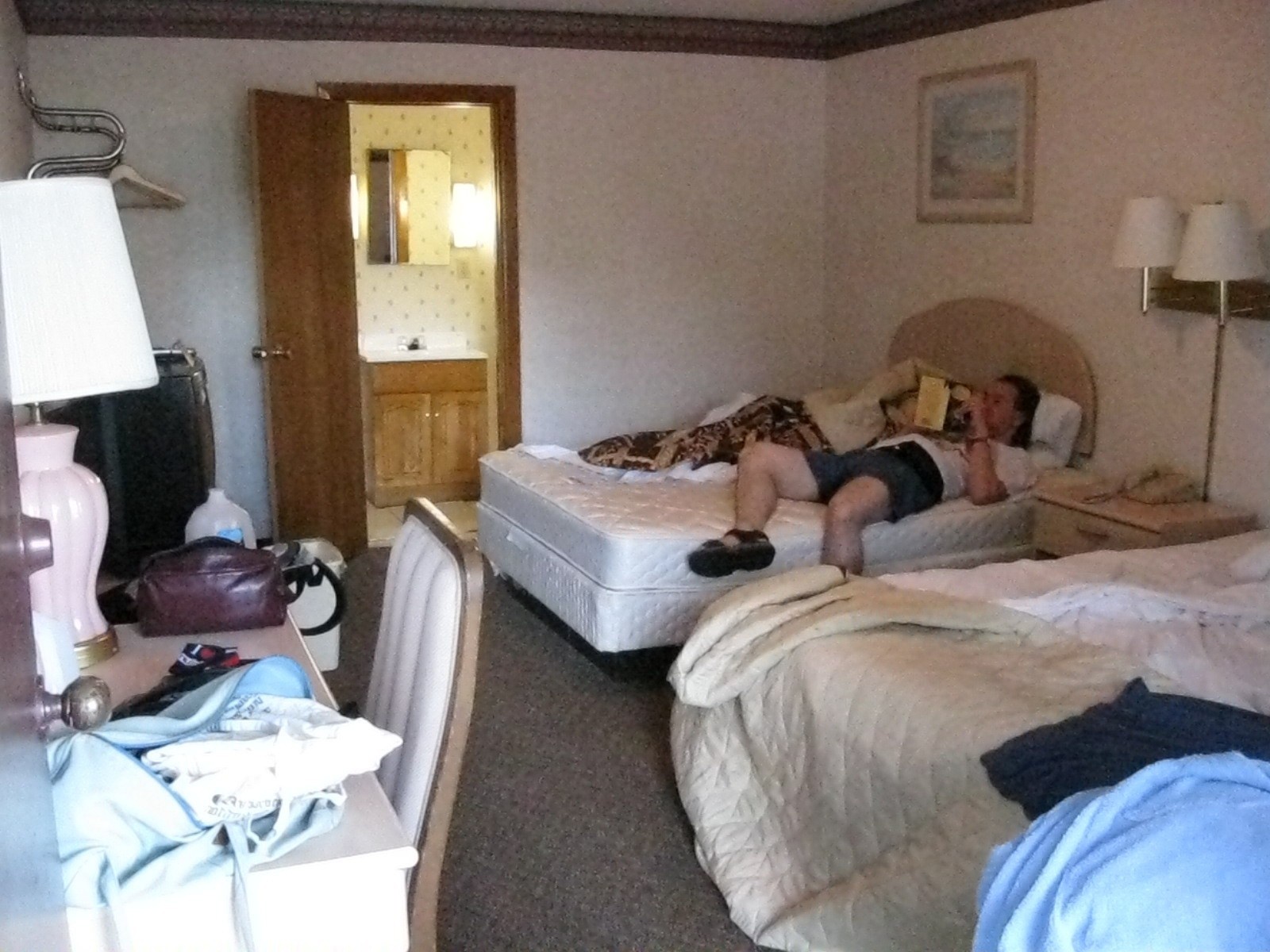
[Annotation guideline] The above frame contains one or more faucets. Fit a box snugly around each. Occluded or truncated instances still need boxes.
[396,336,428,352]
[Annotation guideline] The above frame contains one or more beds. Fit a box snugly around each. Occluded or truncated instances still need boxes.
[480,294,1095,653]
[670,524,1270,952]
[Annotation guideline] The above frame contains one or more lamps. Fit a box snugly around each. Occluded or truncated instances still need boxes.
[1171,200,1268,325]
[1110,193,1194,315]
[0,175,160,668]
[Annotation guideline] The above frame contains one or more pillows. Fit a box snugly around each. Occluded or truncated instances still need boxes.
[1025,392,1083,470]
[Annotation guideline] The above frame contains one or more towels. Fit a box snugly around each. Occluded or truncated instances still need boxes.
[967,745,1270,951]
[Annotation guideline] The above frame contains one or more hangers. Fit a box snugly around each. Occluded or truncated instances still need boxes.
[109,148,186,209]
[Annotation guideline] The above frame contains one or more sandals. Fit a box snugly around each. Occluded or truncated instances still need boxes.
[688,528,778,578]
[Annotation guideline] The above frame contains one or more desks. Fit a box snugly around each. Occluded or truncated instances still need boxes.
[68,581,422,952]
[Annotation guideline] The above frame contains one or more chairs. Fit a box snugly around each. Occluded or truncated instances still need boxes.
[361,496,484,951]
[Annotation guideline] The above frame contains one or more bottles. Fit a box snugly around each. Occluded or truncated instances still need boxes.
[185,486,258,553]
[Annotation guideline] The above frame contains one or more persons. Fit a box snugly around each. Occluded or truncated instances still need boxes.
[689,373,1042,578]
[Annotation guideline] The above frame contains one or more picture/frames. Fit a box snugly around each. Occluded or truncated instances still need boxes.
[914,58,1036,224]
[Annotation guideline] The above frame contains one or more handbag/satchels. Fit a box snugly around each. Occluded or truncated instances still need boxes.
[134,536,287,640]
[47,654,349,952]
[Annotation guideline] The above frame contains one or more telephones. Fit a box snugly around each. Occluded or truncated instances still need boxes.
[1120,464,1201,505]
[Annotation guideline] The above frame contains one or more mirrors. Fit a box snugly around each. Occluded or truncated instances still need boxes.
[350,148,450,265]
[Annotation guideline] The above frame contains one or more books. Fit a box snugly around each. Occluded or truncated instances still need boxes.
[911,374,974,435]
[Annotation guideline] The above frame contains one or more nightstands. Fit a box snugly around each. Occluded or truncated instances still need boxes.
[1028,479,1256,562]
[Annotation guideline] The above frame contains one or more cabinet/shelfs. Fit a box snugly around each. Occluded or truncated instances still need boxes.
[362,363,488,508]
